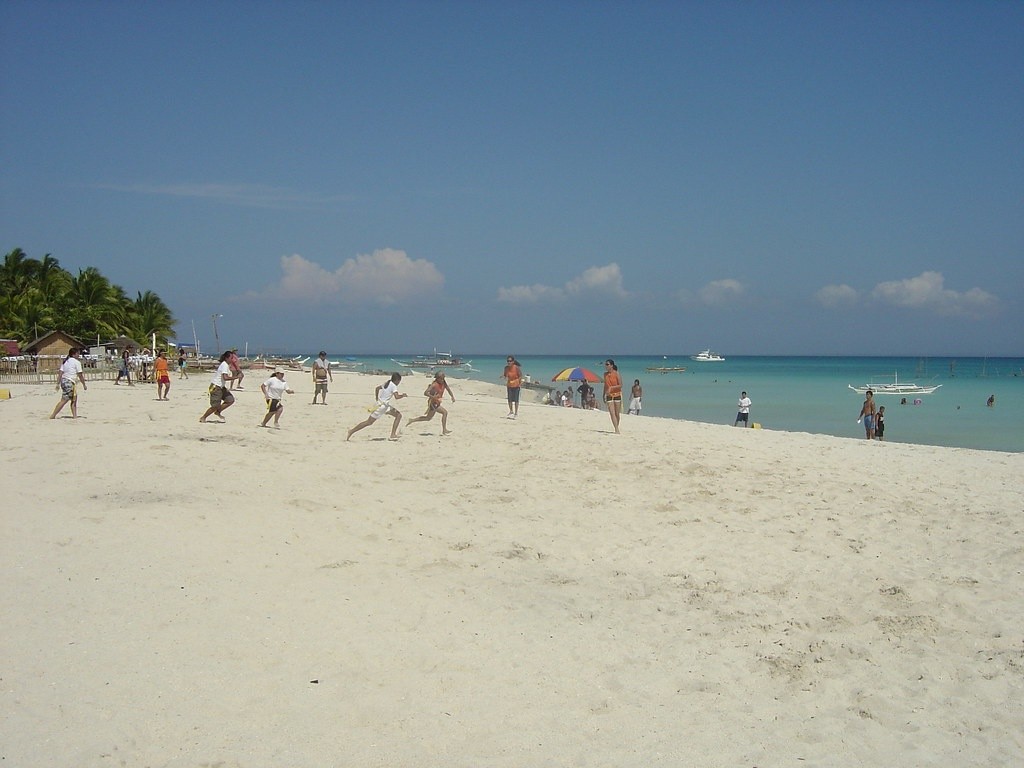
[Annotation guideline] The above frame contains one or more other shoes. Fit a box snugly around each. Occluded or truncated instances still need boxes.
[115,382,119,385]
[129,383,134,386]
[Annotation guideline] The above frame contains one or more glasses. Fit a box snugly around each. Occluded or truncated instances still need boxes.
[606,364,609,366]
[234,350,238,351]
[507,361,511,362]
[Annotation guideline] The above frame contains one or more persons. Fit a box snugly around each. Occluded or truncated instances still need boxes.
[735,392,752,427]
[499,356,522,420]
[603,359,623,434]
[155,349,171,401]
[857,391,876,439]
[347,373,402,443]
[312,351,333,405]
[553,380,595,410]
[136,347,150,380]
[49,348,87,419]
[178,349,188,380]
[627,379,642,415]
[114,345,137,387]
[901,398,907,405]
[260,368,294,426]
[230,347,245,391]
[875,406,886,441]
[406,371,455,434]
[200,351,235,422]
[987,395,995,407]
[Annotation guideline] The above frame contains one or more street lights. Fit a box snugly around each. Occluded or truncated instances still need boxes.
[211,314,224,353]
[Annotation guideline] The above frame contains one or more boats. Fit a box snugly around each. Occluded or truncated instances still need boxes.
[390,345,473,367]
[329,362,363,368]
[409,368,471,382]
[690,349,725,361]
[186,319,311,369]
[500,374,558,390]
[647,366,686,372]
[848,370,943,395]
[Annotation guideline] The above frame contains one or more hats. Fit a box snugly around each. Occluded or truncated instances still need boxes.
[275,367,286,374]
[319,351,327,355]
[128,344,134,348]
[159,349,168,354]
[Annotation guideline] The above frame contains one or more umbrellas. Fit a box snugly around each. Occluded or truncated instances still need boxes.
[551,366,604,408]
